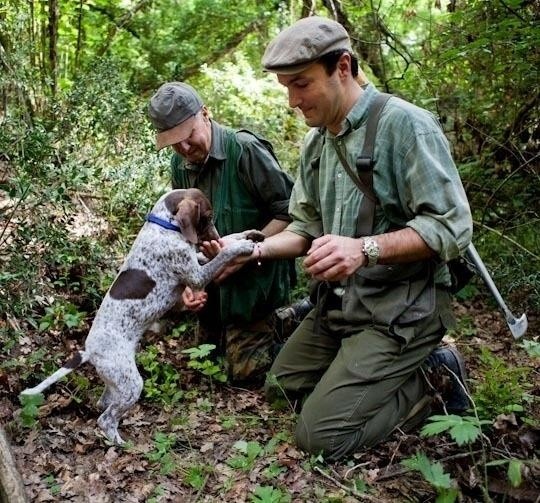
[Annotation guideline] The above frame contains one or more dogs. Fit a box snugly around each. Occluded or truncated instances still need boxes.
[18,187,266,447]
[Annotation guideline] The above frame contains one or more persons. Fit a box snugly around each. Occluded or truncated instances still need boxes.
[199,15,477,463]
[143,81,297,388]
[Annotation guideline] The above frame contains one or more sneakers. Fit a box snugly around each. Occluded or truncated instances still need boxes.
[294,297,313,319]
[423,344,473,422]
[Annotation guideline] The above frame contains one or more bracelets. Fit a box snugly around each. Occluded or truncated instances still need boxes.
[254,241,263,266]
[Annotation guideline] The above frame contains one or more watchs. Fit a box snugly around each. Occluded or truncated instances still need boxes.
[359,234,380,267]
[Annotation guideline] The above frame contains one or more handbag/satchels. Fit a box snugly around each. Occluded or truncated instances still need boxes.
[434,251,478,297]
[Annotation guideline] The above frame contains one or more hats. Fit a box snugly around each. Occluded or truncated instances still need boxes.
[144,80,206,154]
[259,14,356,76]
